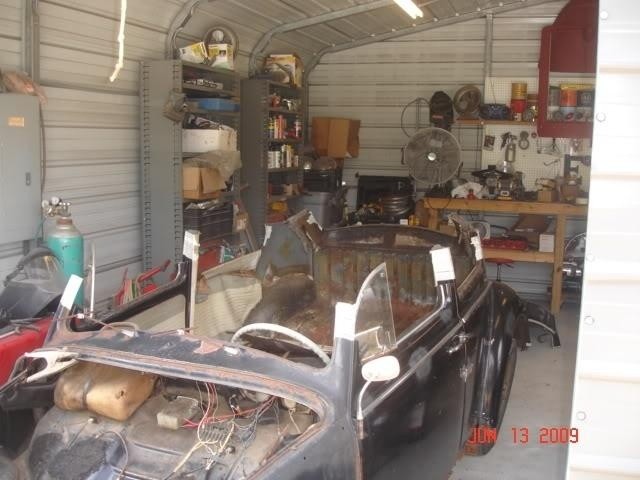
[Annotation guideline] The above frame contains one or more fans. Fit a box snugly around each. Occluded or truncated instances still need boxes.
[404,125,462,187]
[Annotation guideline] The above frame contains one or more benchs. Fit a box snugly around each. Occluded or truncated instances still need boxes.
[241,245,441,356]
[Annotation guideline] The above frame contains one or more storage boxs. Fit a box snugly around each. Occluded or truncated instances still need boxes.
[178,41,208,64]
[183,164,228,200]
[304,190,333,229]
[208,43,235,71]
[183,126,236,154]
[311,115,362,159]
[266,54,305,85]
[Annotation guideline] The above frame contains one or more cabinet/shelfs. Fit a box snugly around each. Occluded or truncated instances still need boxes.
[242,76,308,252]
[136,62,244,280]
[422,197,588,316]
[538,26,597,137]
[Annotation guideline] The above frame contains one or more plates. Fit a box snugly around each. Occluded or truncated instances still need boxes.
[453,84,480,113]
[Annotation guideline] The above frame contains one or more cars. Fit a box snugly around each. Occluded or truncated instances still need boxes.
[5,221,524,479]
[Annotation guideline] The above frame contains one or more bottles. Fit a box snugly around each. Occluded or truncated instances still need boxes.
[486,172,497,199]
[511,81,594,119]
[267,115,302,171]
